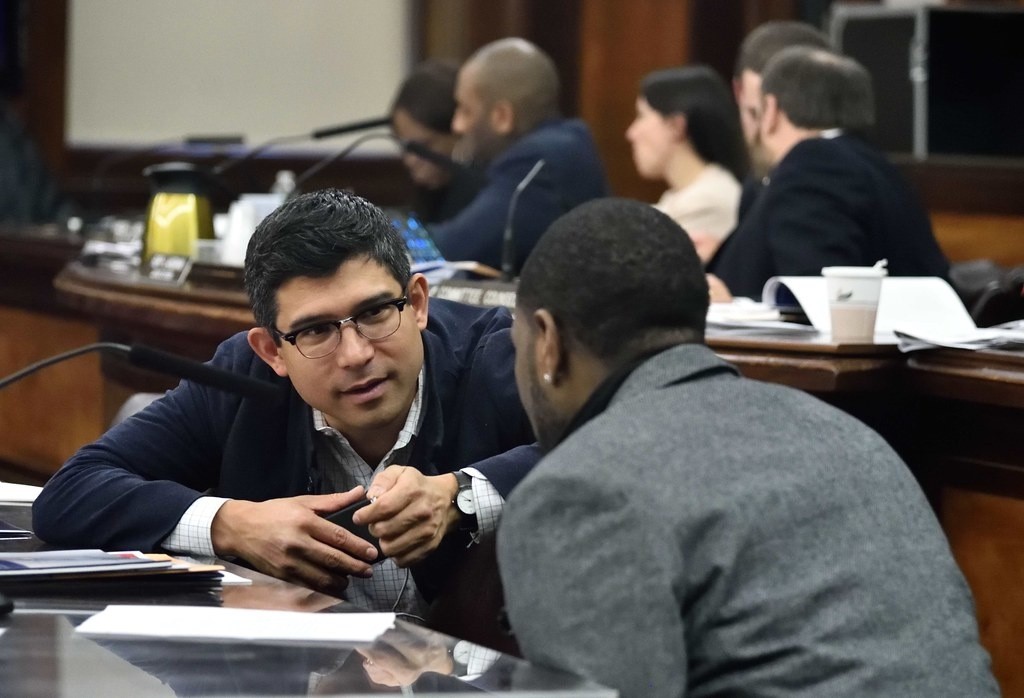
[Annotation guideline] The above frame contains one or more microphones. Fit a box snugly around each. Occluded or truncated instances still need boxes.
[0,343,280,405]
[197,111,546,284]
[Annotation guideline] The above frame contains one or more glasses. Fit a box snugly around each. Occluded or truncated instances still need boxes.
[274,286,409,360]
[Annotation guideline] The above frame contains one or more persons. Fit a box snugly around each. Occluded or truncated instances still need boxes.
[392,38,605,277]
[625,24,944,301]
[32,191,546,656]
[496,199,1000,698]
[73,565,519,698]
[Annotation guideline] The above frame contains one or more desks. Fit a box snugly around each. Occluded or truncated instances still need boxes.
[0,213,1024,698]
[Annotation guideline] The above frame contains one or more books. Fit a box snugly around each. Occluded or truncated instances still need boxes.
[409,261,504,279]
[706,276,975,342]
[1,550,224,575]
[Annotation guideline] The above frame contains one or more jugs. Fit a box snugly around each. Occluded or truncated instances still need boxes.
[141,162,213,267]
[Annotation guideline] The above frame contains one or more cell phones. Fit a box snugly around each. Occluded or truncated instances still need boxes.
[324,498,387,568]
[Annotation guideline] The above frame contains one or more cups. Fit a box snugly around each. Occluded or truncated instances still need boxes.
[823,267,887,343]
